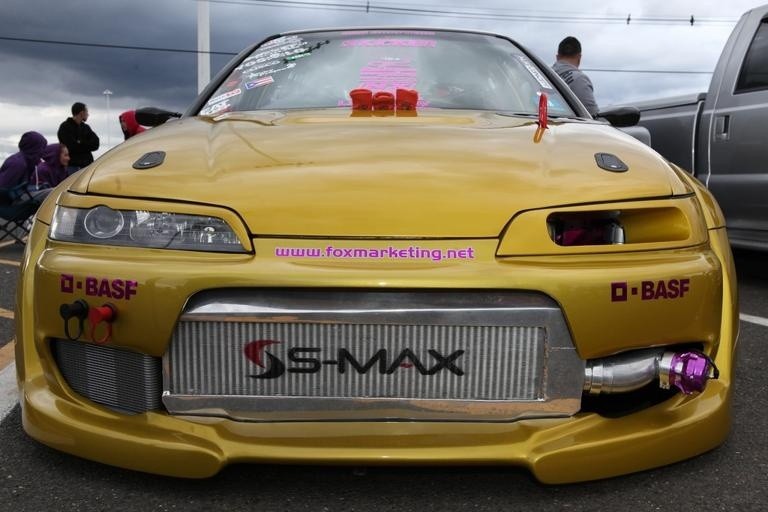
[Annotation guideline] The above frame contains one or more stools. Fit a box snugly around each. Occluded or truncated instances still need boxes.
[0,203,41,246]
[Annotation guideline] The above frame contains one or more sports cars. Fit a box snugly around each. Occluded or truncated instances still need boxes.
[13,29,740,486]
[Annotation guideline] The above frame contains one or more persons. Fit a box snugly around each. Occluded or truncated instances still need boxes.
[31,143,69,189]
[58,101,99,170]
[0,131,59,223]
[543,35,599,116]
[118,111,146,141]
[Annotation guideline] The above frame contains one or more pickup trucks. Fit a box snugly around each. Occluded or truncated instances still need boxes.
[596,6,766,251]
